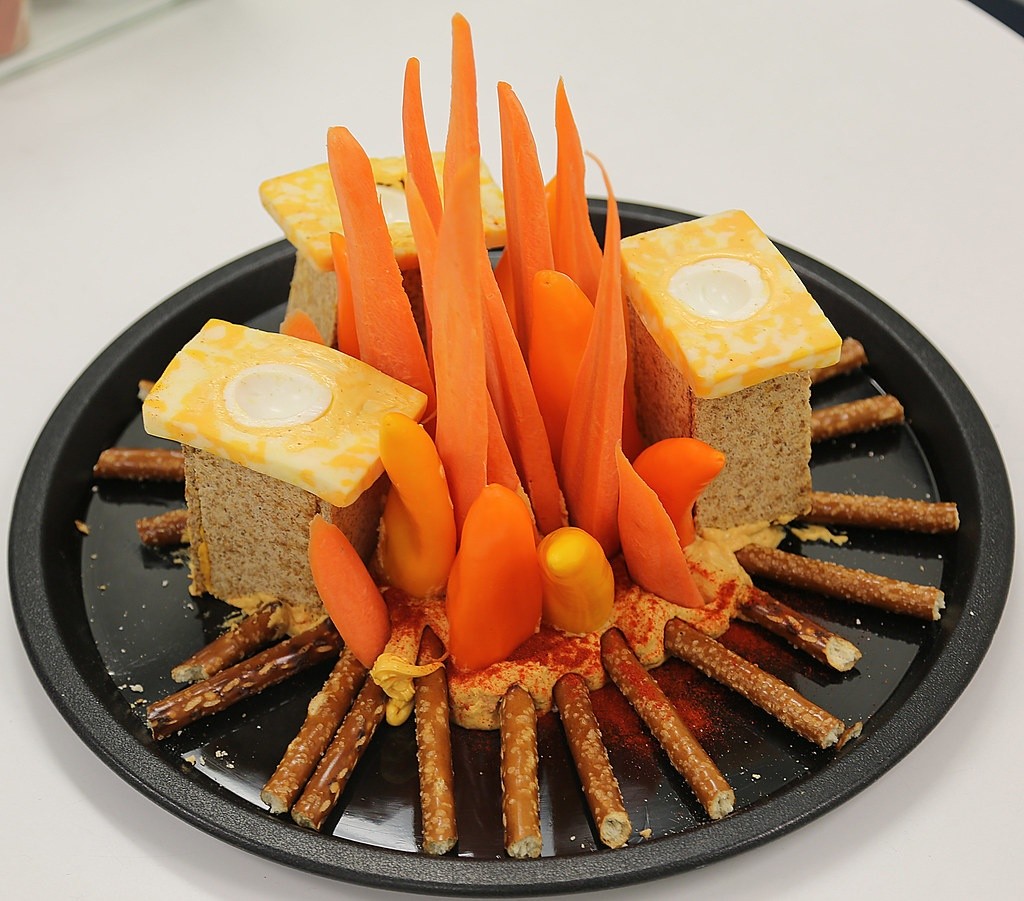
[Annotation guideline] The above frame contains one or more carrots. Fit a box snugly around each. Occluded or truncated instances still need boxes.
[286,10,724,662]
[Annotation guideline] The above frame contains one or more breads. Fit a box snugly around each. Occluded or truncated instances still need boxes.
[176,436,385,633]
[623,303,816,537]
[284,250,431,355]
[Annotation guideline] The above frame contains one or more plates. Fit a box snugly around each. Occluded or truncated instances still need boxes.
[7,197,1013,898]
